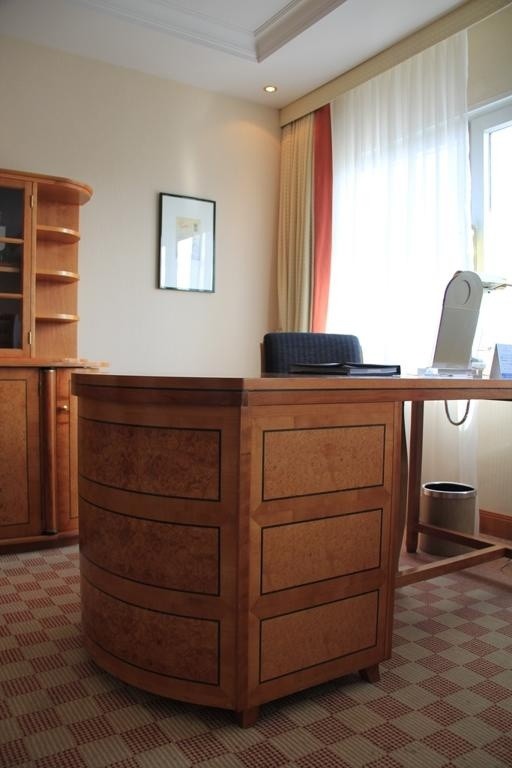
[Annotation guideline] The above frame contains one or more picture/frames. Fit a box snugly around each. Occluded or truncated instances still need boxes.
[158,192,217,294]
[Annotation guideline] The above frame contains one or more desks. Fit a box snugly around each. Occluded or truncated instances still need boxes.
[72,369,510,729]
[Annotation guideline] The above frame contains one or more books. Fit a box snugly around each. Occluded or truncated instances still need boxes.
[289,361,401,377]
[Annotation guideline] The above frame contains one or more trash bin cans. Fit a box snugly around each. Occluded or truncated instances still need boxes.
[420,482,479,556]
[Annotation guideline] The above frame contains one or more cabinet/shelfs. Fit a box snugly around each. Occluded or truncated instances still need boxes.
[0,167,99,544]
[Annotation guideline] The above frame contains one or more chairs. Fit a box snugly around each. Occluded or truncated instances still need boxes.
[259,333,364,374]
